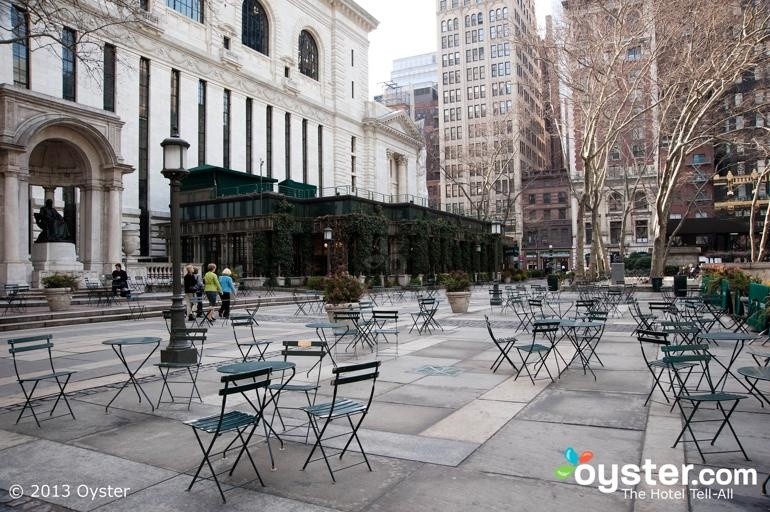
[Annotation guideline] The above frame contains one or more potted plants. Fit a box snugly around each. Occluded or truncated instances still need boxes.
[444,271,471,313]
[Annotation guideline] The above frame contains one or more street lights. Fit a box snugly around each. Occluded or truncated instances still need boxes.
[475,243,483,285]
[548,244,554,269]
[489,215,504,306]
[323,225,333,276]
[160,131,199,367]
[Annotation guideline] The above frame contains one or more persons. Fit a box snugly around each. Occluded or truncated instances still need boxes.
[110,263,128,297]
[183,263,237,321]
[33,199,71,242]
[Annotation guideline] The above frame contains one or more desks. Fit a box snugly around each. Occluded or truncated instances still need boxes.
[216,362,296,459]
[102,337,160,412]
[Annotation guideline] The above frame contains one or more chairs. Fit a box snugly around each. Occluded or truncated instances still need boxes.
[265,341,326,446]
[299,361,381,483]
[484,279,770,488]
[0,274,444,362]
[153,329,208,409]
[7,334,78,426]
[182,367,272,504]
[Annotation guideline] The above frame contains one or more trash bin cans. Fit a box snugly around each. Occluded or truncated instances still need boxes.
[652,278,663,293]
[547,274,559,291]
[674,275,687,296]
